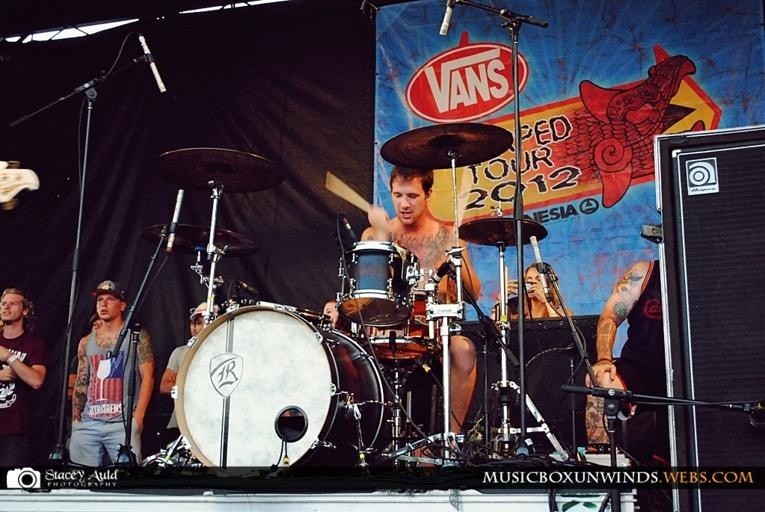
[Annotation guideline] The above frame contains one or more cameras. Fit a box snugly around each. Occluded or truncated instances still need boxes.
[513,282,534,294]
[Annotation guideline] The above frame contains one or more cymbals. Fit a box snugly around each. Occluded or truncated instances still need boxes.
[141,223,257,255]
[159,146,283,194]
[456,218,547,245]
[380,123,513,170]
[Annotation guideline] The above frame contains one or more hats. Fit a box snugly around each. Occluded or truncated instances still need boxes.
[190,302,220,322]
[89,281,129,302]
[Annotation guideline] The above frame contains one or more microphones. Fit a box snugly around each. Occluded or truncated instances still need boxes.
[343,217,359,242]
[165,189,185,252]
[529,236,550,302]
[139,35,166,94]
[439,0,456,37]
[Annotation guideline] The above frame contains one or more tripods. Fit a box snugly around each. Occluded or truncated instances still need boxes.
[374,243,569,475]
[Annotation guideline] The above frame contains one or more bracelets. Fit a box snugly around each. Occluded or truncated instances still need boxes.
[496,297,501,302]
[6,353,18,365]
[597,356,612,363]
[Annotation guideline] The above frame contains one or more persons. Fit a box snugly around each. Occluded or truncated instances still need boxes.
[66,279,158,470]
[62,312,106,458]
[320,292,351,336]
[333,162,480,472]
[490,262,576,321]
[157,300,218,456]
[0,287,50,468]
[582,257,674,511]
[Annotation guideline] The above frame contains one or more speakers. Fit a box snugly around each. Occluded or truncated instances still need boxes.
[652,123,765,512]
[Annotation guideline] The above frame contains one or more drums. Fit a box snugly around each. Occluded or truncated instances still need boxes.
[170,304,392,475]
[338,241,420,328]
[256,300,332,325]
[354,288,442,363]
